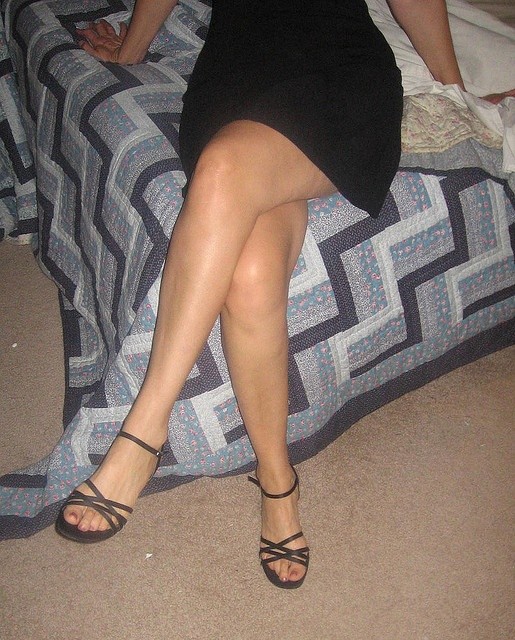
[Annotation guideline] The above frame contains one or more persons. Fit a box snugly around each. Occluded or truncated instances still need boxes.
[55,0,466,589]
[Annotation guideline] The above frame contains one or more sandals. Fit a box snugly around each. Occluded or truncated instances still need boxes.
[248,458,309,589]
[55,431,165,543]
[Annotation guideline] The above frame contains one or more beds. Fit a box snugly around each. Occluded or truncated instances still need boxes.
[0,1,515,544]
